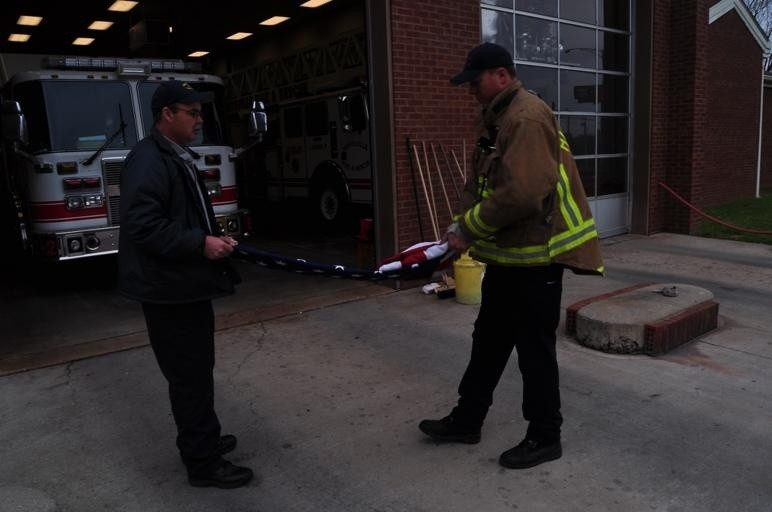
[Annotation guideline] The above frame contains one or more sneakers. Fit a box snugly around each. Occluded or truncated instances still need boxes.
[183,457,254,488]
[414,415,485,444]
[498,439,565,470]
[217,432,241,455]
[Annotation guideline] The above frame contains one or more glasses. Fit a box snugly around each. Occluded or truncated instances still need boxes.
[171,106,202,119]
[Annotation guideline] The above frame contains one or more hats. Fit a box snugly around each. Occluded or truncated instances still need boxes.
[150,80,216,104]
[448,41,514,85]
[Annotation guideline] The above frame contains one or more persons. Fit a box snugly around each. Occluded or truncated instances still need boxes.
[116,81,254,489]
[418,42,604,469]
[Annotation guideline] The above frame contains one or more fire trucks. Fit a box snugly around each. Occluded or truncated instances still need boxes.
[229,82,374,228]
[0,57,242,262]
